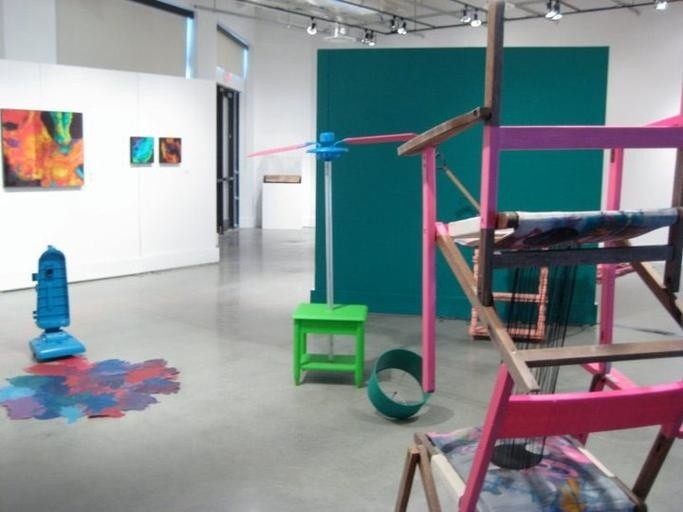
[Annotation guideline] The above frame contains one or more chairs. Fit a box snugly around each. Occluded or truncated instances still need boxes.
[395,0,683,512]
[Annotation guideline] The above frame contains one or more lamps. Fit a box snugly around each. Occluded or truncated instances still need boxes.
[306,0,667,35]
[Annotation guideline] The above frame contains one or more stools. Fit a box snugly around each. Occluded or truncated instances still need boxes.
[292,303,368,386]
